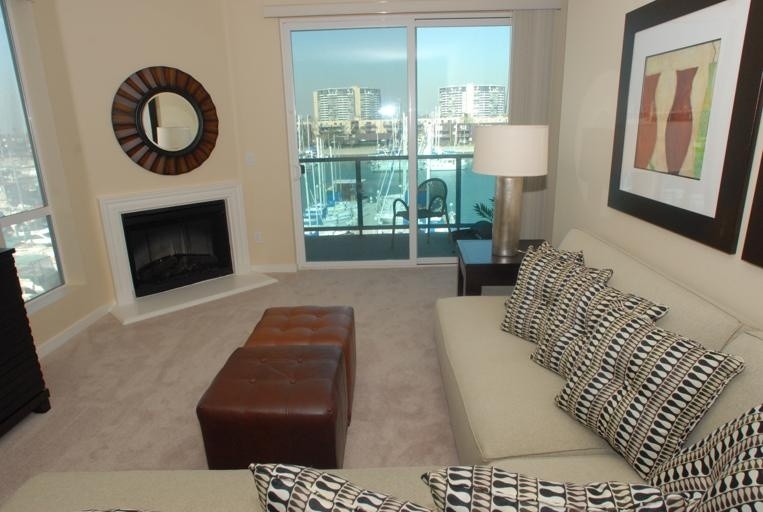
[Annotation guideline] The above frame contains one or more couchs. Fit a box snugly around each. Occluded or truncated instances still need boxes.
[2,458,763,511]
[243,306,356,423]
[434,293,511,464]
[194,343,349,470]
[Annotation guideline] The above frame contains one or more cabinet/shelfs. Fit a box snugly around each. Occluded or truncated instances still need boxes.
[0,249,53,438]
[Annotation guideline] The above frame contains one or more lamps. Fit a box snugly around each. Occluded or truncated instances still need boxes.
[471,124,548,257]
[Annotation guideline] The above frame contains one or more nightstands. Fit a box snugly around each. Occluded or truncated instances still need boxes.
[456,239,546,296]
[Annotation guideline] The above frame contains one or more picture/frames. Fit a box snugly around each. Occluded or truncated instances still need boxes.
[742,149,763,269]
[609,1,763,254]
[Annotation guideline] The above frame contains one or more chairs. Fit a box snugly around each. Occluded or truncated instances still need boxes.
[391,178,452,250]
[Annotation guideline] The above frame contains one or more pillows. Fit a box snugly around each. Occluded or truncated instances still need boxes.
[648,405,763,511]
[535,241,584,265]
[421,465,706,511]
[530,280,671,380]
[249,463,433,512]
[500,245,614,344]
[554,313,744,482]
